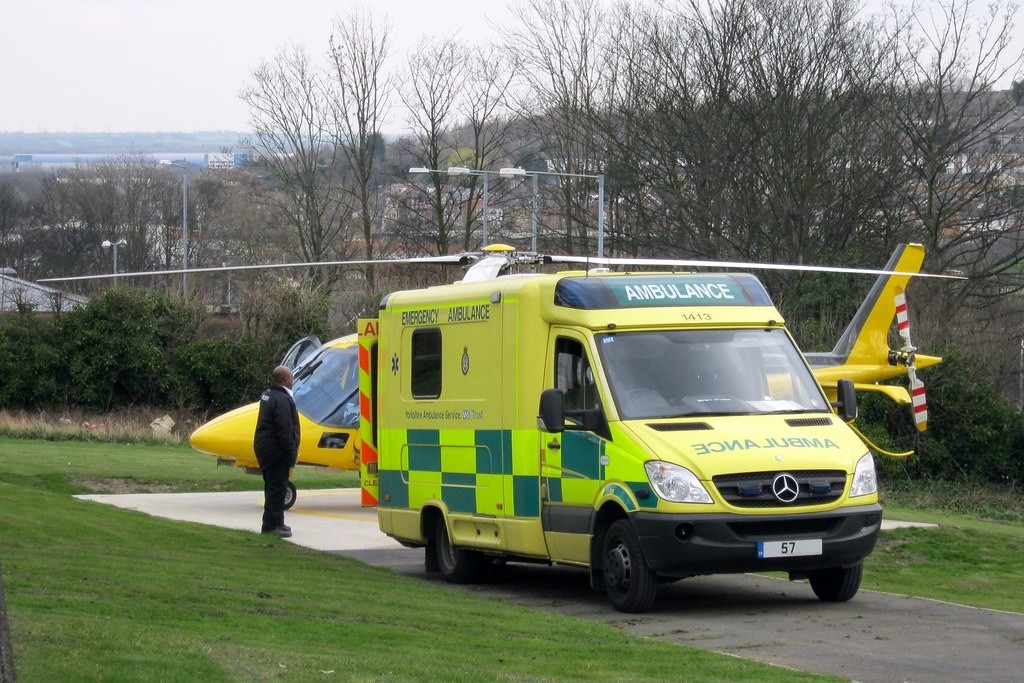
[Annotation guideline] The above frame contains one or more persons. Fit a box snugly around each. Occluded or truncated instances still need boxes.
[254,366,301,537]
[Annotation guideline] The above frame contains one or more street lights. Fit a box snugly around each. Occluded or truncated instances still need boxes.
[498,166,605,271]
[409,166,488,257]
[448,165,538,274]
[158,159,187,303]
[101,238,128,289]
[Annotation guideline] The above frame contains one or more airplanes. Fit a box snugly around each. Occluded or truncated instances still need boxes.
[37,243,942,541]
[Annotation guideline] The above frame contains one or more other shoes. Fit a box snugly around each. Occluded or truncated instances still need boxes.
[261,527,292,537]
[279,524,291,531]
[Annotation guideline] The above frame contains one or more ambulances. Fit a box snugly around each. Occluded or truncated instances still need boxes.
[354,271,880,619]
